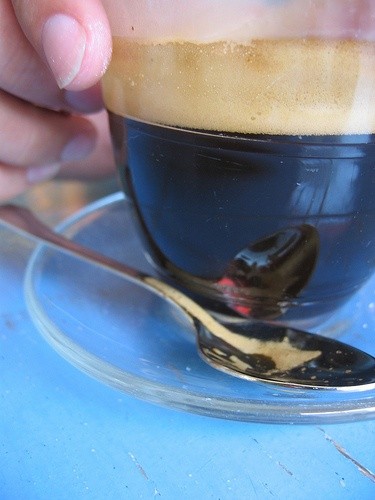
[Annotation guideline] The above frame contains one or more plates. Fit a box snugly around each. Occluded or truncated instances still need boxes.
[23,192,375,426]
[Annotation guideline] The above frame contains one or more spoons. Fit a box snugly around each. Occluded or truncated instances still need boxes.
[0,200,375,393]
[148,223,321,323]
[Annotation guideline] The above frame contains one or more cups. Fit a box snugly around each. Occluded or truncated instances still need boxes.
[100,0,375,333]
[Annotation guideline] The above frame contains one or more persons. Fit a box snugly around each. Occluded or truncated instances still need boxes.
[0,0,375,200]
